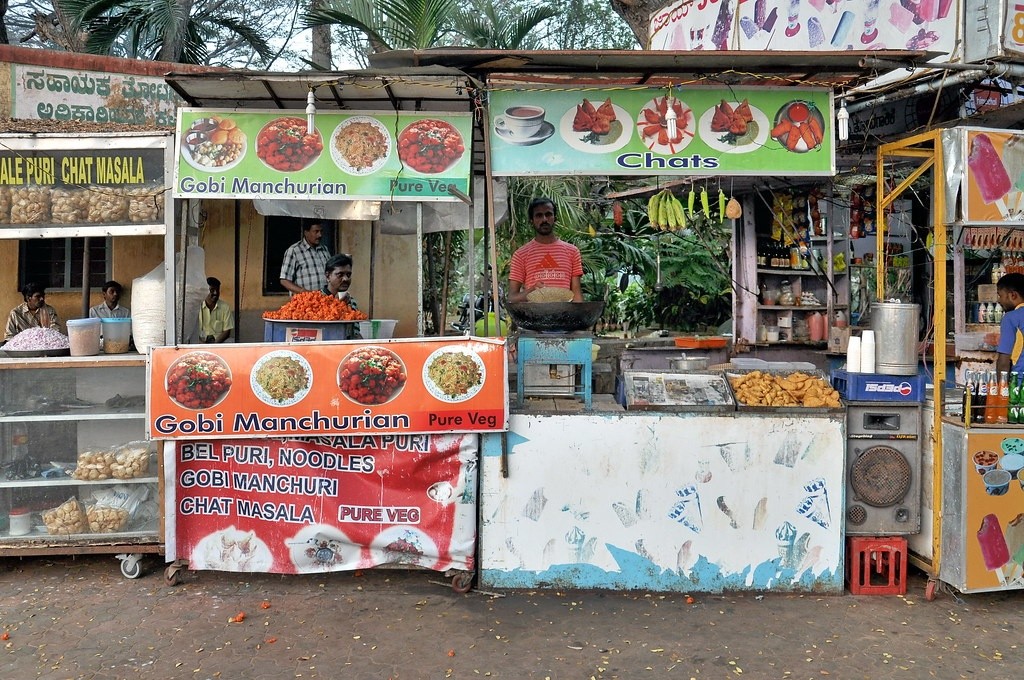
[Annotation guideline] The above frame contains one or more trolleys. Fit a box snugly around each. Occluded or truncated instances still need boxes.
[875,126,1024,603]
[158,68,477,595]
[0,131,166,580]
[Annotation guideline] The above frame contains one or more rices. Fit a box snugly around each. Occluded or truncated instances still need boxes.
[257,357,309,399]
[428,352,482,398]
[334,122,387,171]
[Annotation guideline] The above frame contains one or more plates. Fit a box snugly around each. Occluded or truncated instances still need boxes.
[421,345,486,404]
[164,350,313,412]
[181,117,464,174]
[493,96,825,154]
[34,525,48,534]
[336,346,407,407]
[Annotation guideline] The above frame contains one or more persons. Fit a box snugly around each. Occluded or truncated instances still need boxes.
[995,273,1024,387]
[316,253,362,340]
[88,281,132,339]
[278,219,332,298]
[506,198,586,303]
[2,282,60,345]
[198,277,234,343]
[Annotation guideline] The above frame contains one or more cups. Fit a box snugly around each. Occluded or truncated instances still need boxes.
[846,336,861,373]
[766,326,779,341]
[861,330,875,373]
[764,291,775,305]
[494,105,545,138]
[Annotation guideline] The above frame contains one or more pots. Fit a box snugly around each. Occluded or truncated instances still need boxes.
[505,301,605,332]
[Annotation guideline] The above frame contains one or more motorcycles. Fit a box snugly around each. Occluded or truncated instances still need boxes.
[450,263,506,336]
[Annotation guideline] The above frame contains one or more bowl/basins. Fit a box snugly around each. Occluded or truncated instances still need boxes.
[190,117,219,135]
[665,357,709,370]
[972,438,1024,496]
[592,344,600,362]
[185,133,208,150]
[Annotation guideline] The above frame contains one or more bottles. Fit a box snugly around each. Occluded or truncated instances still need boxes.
[962,371,1024,424]
[991,252,1024,284]
[991,234,1022,247]
[757,241,801,271]
[966,233,988,246]
[850,241,855,264]
[757,325,767,341]
[986,301,994,323]
[994,301,1002,323]
[977,301,986,323]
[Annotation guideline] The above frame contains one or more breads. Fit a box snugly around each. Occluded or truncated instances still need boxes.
[211,116,246,144]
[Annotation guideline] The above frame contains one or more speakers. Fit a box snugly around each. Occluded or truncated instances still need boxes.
[840,398,922,536]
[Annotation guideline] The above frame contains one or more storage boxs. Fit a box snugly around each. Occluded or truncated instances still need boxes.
[845,535,907,595]
[829,368,927,404]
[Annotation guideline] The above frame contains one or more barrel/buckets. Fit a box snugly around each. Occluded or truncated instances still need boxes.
[65,316,132,356]
[869,302,920,375]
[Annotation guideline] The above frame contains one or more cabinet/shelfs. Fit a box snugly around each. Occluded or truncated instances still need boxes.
[0,350,166,580]
[735,194,852,355]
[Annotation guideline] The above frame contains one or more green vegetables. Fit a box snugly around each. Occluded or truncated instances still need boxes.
[580,132,601,144]
[718,131,738,147]
[357,356,388,390]
[414,126,447,162]
[185,359,213,394]
[274,126,306,157]
